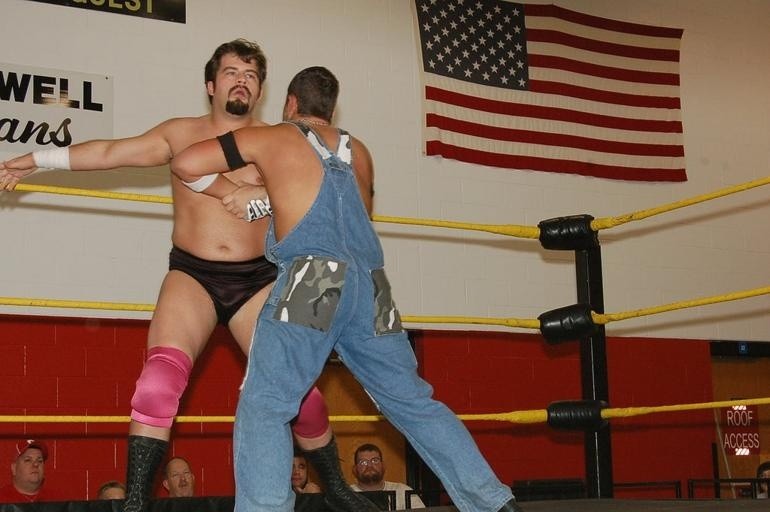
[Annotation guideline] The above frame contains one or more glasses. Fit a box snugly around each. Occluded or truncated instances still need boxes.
[357,457,379,465]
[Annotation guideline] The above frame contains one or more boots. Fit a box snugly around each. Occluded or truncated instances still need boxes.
[298,434,383,512]
[119,435,168,512]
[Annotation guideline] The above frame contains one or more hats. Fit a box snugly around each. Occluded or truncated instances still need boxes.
[13,440,49,463]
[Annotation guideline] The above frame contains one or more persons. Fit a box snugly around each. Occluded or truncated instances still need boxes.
[170,65,520,510]
[1,39,385,510]
[289,445,321,494]
[1,437,50,501]
[348,442,427,511]
[99,480,127,499]
[164,455,195,497]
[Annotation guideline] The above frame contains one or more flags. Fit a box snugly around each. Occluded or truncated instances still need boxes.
[411,0,688,183]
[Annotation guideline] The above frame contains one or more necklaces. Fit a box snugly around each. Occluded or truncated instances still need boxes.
[299,118,330,128]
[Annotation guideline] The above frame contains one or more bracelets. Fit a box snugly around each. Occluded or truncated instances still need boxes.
[179,172,222,192]
[32,145,70,171]
